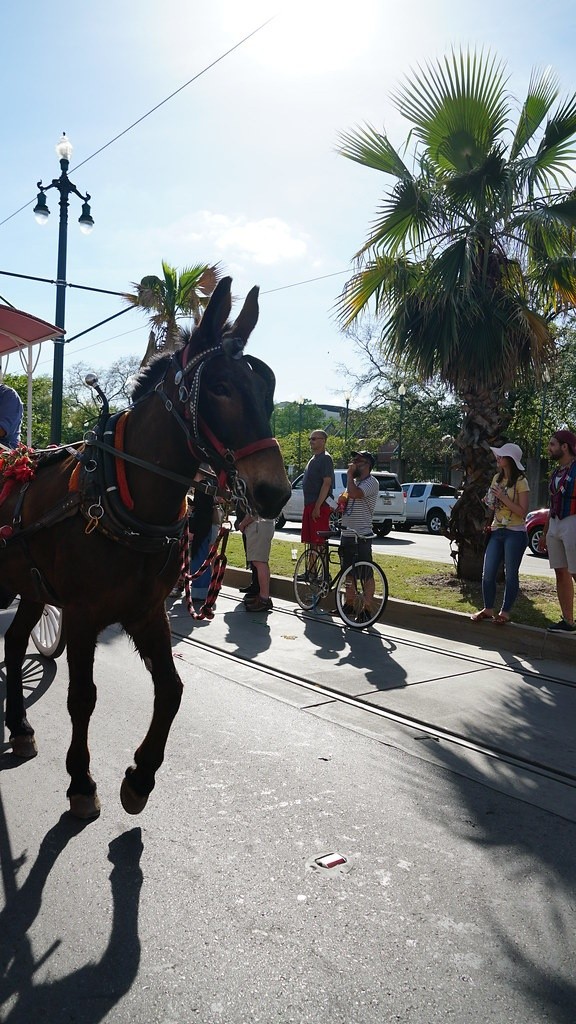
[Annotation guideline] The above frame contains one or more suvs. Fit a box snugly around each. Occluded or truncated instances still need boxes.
[274,469,407,537]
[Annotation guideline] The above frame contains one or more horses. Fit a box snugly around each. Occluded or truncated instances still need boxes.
[0,276,292,821]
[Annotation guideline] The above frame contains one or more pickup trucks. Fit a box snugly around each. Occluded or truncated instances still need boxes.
[394,481,463,536]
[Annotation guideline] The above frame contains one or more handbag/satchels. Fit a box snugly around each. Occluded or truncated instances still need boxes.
[212,503,226,523]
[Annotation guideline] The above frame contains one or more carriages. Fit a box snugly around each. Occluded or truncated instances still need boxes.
[0,275,294,822]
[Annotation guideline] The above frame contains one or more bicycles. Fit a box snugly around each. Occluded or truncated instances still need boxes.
[293,524,389,629]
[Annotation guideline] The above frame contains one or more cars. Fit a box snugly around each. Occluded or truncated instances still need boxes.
[525,508,551,558]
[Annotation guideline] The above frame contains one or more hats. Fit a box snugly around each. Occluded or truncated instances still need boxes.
[351,450,376,467]
[551,431,576,454]
[489,443,526,471]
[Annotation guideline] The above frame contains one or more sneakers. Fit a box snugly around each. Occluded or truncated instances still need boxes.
[245,595,273,612]
[243,597,258,606]
[547,616,576,635]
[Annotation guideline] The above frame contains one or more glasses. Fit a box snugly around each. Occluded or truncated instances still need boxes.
[307,437,326,441]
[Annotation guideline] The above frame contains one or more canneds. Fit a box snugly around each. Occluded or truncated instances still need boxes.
[487,487,496,503]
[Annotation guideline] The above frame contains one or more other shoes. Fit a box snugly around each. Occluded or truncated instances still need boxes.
[352,609,372,624]
[293,572,311,582]
[245,588,261,597]
[239,584,259,592]
[309,572,333,585]
[328,603,356,618]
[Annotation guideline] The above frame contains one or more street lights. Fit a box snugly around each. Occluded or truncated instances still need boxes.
[30,130,98,447]
[84,419,90,438]
[343,391,351,442]
[296,394,305,469]
[67,421,73,444]
[397,383,407,484]
[535,369,551,460]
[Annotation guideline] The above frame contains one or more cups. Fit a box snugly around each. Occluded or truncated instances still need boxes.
[291,549,298,560]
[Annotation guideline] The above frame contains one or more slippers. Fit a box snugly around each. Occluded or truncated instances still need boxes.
[493,614,512,624]
[471,611,495,620]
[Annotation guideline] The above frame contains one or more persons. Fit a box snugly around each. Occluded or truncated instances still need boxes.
[292,430,334,587]
[234,503,275,612]
[471,443,530,624]
[167,462,224,603]
[538,431,576,635]
[0,365,23,459]
[329,451,379,623]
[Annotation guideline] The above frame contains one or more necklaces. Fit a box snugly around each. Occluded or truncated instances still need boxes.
[344,480,361,516]
[494,480,516,523]
[548,459,574,496]
